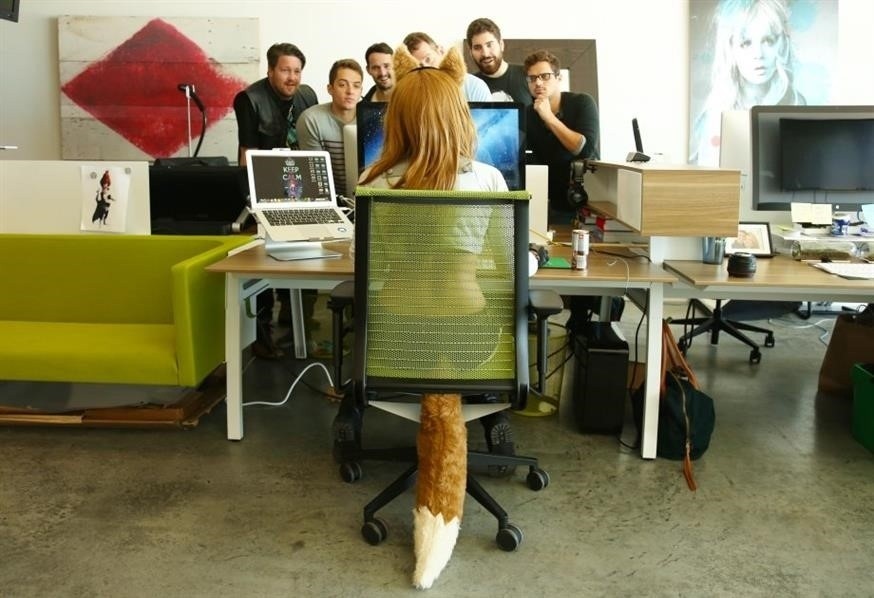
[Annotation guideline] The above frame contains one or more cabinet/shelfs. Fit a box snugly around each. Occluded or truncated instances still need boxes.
[584,159,741,239]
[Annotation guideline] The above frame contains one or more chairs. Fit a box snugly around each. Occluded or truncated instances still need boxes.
[667,298,775,365]
[324,186,564,552]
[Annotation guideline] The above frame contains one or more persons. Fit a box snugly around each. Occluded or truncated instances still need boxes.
[731,230,759,249]
[706,0,807,110]
[467,18,533,106]
[358,43,515,380]
[296,59,364,203]
[403,32,494,104]
[526,49,599,204]
[358,42,395,105]
[234,43,318,167]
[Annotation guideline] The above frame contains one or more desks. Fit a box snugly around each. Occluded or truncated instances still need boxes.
[204,221,874,460]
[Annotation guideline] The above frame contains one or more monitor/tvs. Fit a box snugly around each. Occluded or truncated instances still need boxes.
[780,118,874,191]
[355,102,526,192]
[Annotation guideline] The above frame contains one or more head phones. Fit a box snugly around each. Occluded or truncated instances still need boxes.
[566,158,588,211]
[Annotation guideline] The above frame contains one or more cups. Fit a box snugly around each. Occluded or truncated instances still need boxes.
[701,237,725,265]
[831,211,850,238]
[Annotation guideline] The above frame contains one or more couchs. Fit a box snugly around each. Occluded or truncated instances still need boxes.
[0,233,259,429]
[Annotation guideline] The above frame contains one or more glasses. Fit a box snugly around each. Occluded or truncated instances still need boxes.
[527,73,553,82]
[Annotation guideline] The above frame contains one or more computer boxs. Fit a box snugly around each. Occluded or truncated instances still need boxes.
[571,320,629,437]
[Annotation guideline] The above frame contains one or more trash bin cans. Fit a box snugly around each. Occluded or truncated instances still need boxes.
[850,363,874,451]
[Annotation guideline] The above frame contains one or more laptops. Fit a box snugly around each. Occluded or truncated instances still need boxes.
[245,149,355,242]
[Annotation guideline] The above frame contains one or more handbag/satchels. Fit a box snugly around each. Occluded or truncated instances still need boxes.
[632,320,715,468]
[817,303,874,396]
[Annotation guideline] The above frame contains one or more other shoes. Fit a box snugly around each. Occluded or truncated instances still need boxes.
[480,402,517,477]
[278,315,319,330]
[253,331,285,361]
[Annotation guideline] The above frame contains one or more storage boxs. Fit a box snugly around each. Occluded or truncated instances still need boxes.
[850,362,874,457]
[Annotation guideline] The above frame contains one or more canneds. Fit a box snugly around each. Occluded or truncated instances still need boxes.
[572,229,590,273]
[829,212,850,236]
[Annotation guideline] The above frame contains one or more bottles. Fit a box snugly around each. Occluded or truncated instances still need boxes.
[572,229,589,270]
